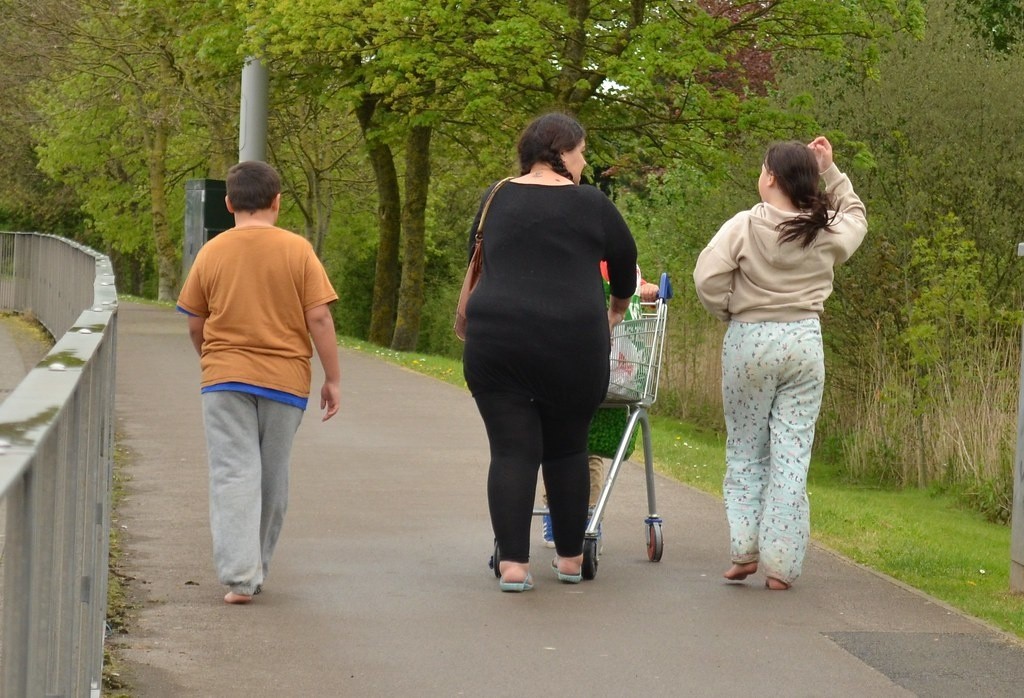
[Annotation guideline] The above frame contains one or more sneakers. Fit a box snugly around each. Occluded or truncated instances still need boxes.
[542,513,556,548]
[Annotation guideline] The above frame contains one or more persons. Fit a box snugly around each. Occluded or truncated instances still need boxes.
[461,114,638,592]
[693,135,872,591]
[175,160,343,604]
[540,257,660,556]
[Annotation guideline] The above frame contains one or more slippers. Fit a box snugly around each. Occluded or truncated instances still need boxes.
[550,558,582,584]
[500,571,533,592]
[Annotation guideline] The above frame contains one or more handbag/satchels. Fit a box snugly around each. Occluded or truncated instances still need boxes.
[453,175,514,341]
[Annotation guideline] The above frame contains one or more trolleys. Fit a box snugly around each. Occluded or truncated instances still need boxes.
[485,270,677,577]
